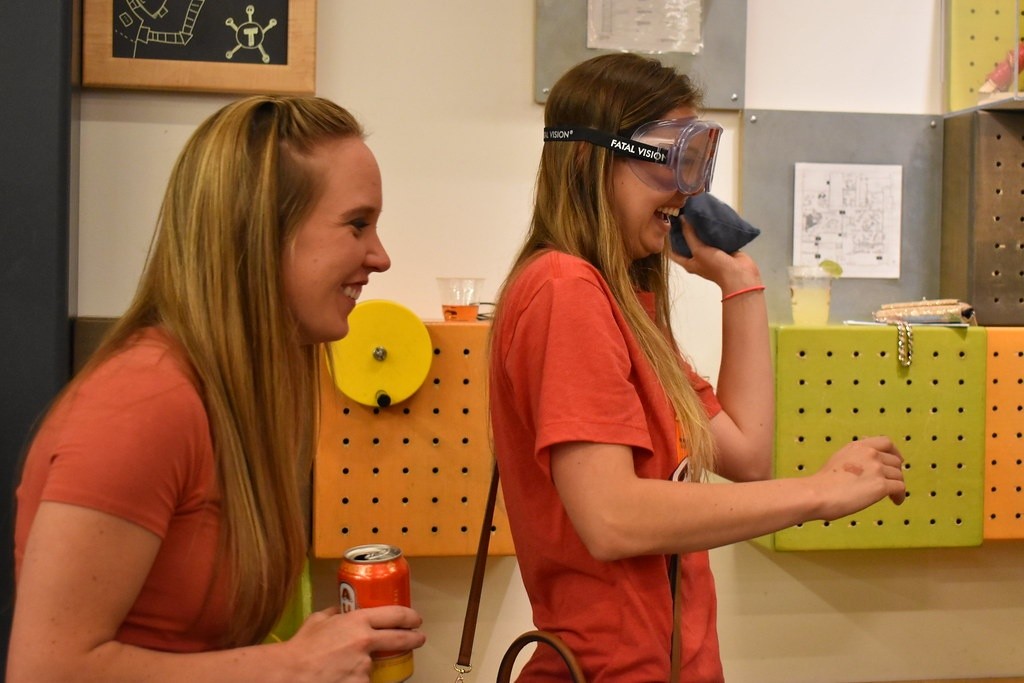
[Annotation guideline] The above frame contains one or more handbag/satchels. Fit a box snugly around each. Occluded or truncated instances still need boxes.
[874,298,977,368]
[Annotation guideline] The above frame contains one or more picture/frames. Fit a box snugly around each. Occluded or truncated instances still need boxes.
[83,0,320,97]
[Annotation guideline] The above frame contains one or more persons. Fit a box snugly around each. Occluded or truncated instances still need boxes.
[6,94,426,683]
[486,53,905,683]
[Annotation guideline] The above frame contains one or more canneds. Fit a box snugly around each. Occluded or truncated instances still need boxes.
[336,543,415,683]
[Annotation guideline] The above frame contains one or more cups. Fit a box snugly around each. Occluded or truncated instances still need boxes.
[439,276,485,321]
[788,265,830,326]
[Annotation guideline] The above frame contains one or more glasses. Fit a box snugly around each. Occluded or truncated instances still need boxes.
[629,117,724,197]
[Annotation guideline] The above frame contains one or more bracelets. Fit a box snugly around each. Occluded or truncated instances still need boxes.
[721,285,765,302]
